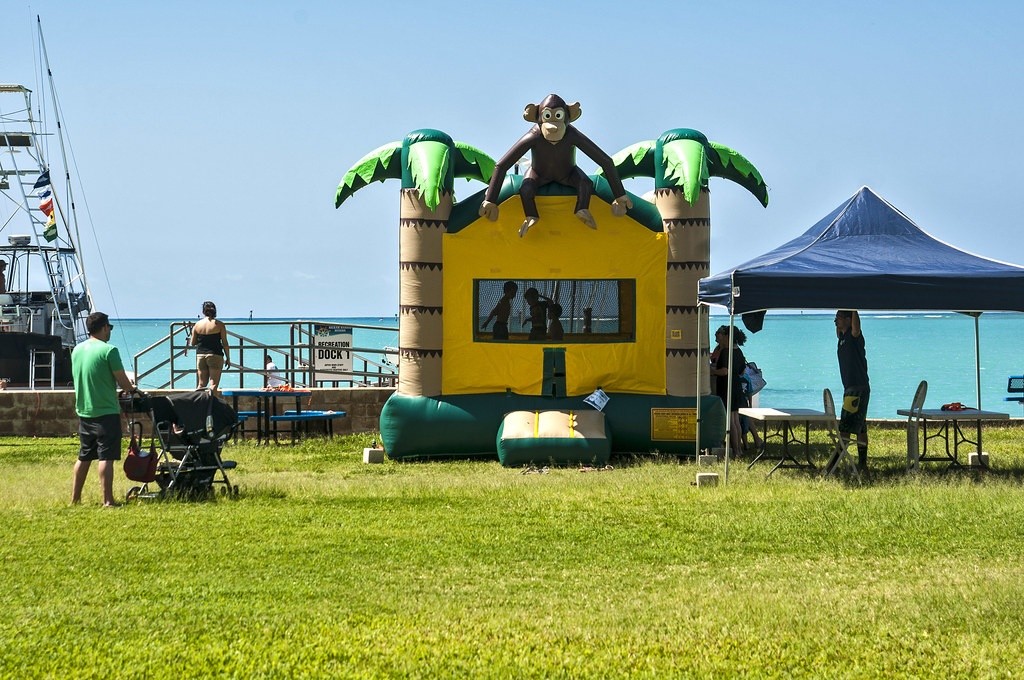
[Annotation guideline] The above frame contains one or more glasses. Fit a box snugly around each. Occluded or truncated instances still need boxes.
[722,332,727,336]
[103,323,113,330]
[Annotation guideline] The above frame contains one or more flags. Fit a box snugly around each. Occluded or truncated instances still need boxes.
[39,198,54,217]
[33,171,50,189]
[43,218,58,243]
[38,184,51,200]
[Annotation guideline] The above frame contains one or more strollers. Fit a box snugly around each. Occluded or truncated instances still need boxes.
[117,387,244,506]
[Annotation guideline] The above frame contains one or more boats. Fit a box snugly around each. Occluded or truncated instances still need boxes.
[0,14,91,390]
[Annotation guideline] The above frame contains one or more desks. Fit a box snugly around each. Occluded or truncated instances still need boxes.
[222,390,312,448]
[897,409,1009,474]
[738,408,835,476]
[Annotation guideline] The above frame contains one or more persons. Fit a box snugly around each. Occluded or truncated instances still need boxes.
[480,281,518,339]
[266,354,282,387]
[191,301,230,396]
[69,312,135,508]
[709,325,763,460]
[0,260,9,294]
[521,288,554,342]
[546,304,564,341]
[829,310,870,476]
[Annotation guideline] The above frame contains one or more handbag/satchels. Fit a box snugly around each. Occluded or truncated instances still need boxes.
[124,439,157,483]
[738,362,766,397]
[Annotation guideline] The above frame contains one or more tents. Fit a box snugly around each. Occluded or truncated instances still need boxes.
[696,185,1023,485]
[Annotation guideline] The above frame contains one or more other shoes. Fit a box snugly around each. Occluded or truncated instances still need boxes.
[756,444,762,449]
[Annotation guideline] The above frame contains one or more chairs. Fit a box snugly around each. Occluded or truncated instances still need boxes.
[907,380,927,470]
[824,389,867,485]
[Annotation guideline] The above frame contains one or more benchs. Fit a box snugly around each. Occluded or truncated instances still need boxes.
[226,410,346,446]
